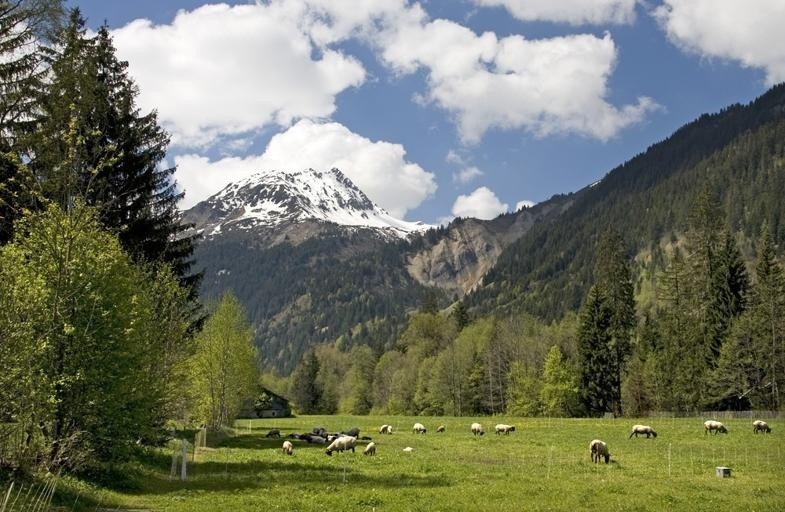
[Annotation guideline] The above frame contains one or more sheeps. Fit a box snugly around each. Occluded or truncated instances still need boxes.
[378,422,515,435]
[325,436,357,456]
[363,442,376,456]
[264,427,372,445]
[282,441,293,456]
[629,425,657,439]
[704,421,728,435]
[589,439,613,464]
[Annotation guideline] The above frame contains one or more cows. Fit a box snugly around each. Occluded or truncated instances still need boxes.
[753,420,772,434]
